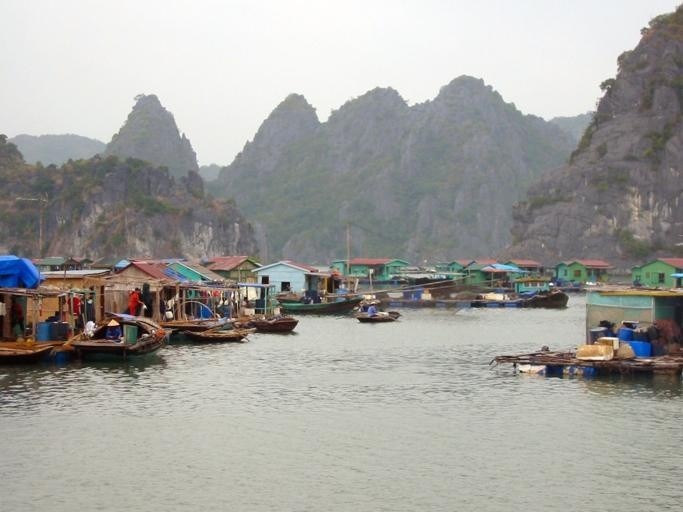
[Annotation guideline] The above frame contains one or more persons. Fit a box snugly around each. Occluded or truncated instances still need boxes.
[104,317,122,342]
[67,292,95,332]
[368,301,379,317]
[10,298,27,341]
[125,287,140,316]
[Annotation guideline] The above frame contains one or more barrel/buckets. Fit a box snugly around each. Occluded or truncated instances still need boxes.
[633,331,650,341]
[50,321,69,340]
[35,321,51,341]
[122,323,139,345]
[588,327,611,345]
[617,326,634,342]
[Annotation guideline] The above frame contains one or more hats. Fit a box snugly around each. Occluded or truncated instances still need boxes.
[108,319,119,328]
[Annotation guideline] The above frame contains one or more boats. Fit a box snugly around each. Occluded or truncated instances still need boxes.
[276,297,362,313]
[356,311,399,322]
[185,328,256,342]
[243,317,298,331]
[379,287,568,308]
[67,310,167,355]
